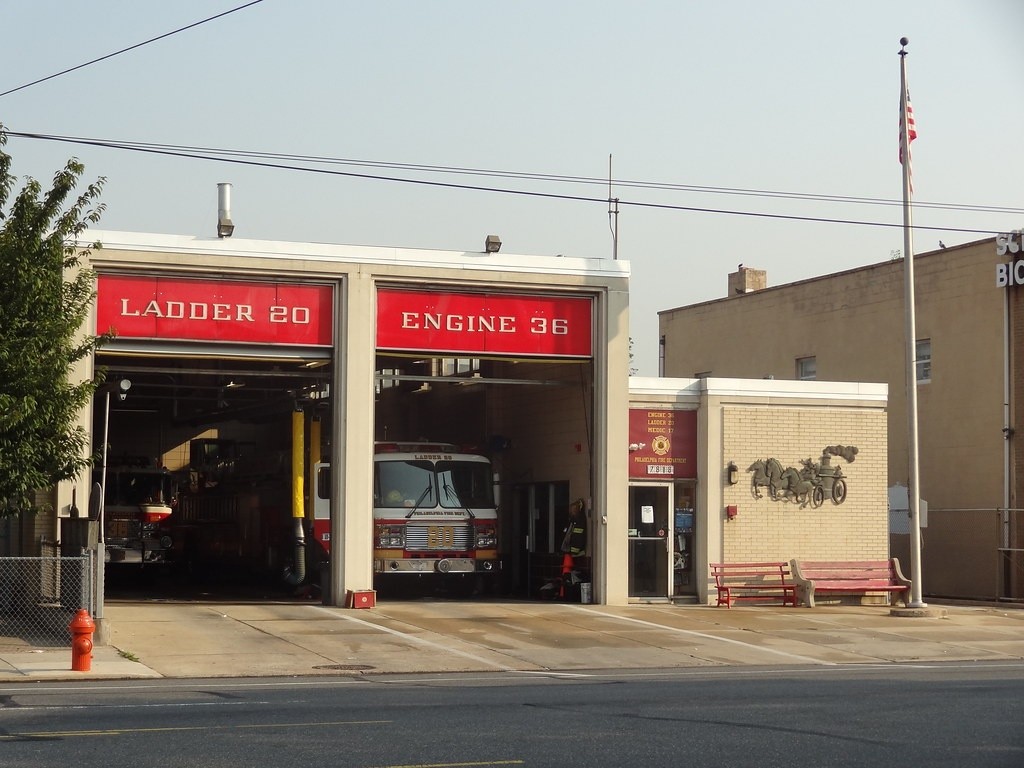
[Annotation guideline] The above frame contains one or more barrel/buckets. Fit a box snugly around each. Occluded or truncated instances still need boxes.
[575,582,591,603]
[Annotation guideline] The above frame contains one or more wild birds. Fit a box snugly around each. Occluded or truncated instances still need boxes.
[938,240,947,249]
[738,263,743,267]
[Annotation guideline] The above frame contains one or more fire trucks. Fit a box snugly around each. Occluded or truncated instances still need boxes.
[91,458,210,592]
[277,435,510,595]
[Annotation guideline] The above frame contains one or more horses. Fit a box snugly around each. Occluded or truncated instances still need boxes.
[749,457,813,508]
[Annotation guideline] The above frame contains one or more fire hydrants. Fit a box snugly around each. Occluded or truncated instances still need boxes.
[67,608,96,672]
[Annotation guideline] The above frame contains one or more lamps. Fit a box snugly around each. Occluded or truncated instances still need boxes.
[484,234,502,254]
[216,218,235,238]
[728,462,740,485]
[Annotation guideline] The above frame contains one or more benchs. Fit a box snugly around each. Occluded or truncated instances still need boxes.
[709,560,801,606]
[790,557,911,609]
